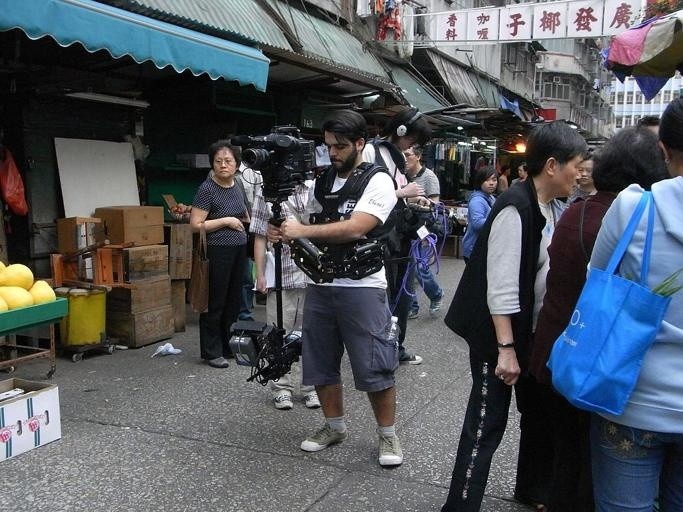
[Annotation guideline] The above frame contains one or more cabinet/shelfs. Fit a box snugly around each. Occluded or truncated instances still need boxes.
[1,295,67,378]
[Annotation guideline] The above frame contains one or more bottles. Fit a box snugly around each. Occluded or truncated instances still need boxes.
[381,316,400,343]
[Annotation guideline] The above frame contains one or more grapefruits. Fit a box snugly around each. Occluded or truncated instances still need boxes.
[0,262,6,273]
[0,297,8,311]
[0,263,34,290]
[29,281,56,304]
[0,287,35,309]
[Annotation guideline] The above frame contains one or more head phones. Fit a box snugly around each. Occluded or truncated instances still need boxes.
[397,111,422,137]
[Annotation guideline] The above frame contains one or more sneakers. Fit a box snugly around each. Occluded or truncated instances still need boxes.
[378,431,403,467]
[304,393,320,408]
[407,310,419,320]
[301,423,347,453]
[429,295,442,316]
[400,351,422,365]
[274,394,293,409]
[206,357,229,368]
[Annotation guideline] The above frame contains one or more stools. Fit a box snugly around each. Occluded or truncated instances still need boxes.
[52,216,114,254]
[47,239,140,301]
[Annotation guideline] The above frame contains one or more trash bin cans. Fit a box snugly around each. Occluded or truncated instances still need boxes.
[56,285,115,362]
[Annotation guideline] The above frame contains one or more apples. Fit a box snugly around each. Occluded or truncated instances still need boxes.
[171,202,192,220]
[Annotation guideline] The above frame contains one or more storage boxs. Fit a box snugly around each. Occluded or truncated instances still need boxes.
[95,205,193,348]
[0,378,61,463]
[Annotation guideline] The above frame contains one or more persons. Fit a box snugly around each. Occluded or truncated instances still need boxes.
[384,135,445,365]
[190,109,403,468]
[444,96,683,512]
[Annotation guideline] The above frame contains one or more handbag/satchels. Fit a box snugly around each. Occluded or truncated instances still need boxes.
[546,192,671,417]
[188,221,209,313]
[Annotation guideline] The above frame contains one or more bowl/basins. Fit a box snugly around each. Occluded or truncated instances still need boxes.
[170,212,190,222]
